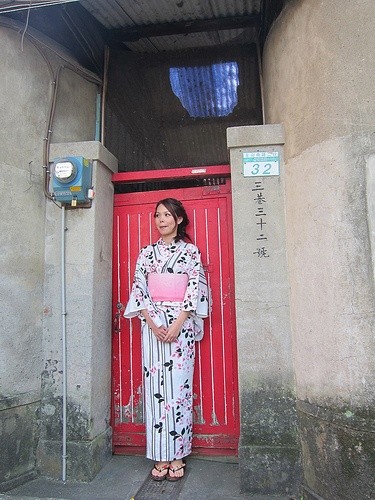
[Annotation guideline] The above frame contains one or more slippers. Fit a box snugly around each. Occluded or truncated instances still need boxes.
[151,464,170,481]
[166,463,186,481]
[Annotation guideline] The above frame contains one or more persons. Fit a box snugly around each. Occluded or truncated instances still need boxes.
[124,198,209,482]
